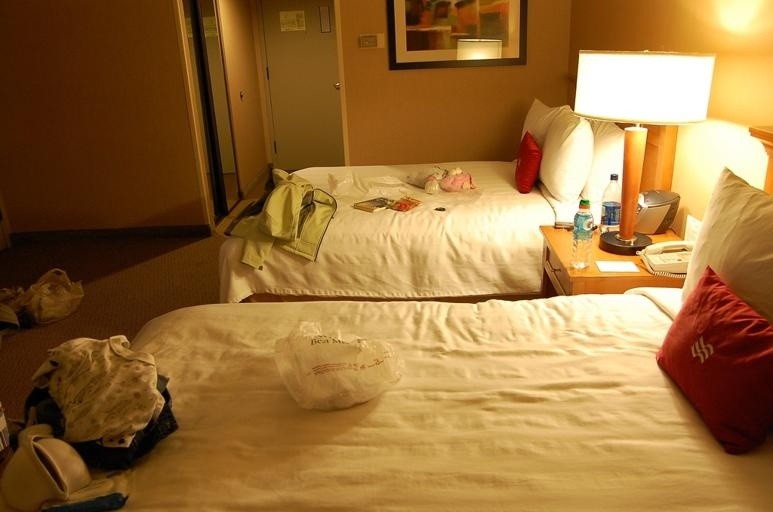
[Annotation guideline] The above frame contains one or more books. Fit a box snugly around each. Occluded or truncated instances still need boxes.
[386,195,422,213]
[354,197,395,213]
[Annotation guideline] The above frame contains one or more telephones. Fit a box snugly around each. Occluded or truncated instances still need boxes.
[644,240,694,274]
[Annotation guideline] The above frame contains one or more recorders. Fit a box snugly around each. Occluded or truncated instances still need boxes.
[634,191,680,235]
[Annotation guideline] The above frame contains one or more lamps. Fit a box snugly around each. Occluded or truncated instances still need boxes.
[569,46,719,260]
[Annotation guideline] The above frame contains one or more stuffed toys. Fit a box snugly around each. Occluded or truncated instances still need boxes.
[405,165,477,195]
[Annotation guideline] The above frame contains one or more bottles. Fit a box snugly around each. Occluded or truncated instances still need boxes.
[571,197,594,270]
[599,173,622,234]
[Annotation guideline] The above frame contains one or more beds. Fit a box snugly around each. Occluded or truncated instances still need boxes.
[216,99,685,304]
[42,125,773,511]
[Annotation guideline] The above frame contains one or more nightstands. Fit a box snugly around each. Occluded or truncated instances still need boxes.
[536,214,706,303]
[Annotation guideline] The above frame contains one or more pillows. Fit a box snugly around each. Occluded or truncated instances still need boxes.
[677,162,773,325]
[649,261,773,457]
[514,95,627,201]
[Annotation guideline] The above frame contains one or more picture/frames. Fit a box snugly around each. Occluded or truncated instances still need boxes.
[384,0,528,70]
